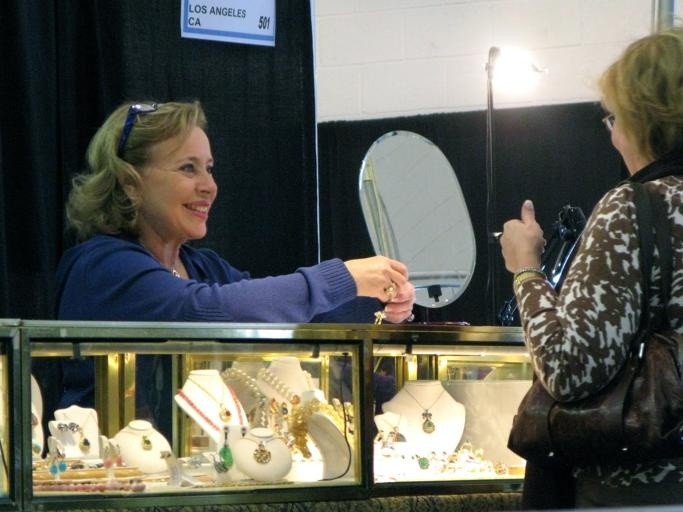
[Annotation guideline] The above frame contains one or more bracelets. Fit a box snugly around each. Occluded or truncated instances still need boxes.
[512,272,542,295]
[513,268,547,280]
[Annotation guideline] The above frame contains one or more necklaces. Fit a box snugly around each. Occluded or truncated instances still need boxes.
[176,364,447,472]
[122,425,157,451]
[62,408,94,453]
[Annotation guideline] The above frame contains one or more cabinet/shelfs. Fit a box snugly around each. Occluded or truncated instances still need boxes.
[1,319,533,511]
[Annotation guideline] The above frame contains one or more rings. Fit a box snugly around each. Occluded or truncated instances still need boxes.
[385,282,396,297]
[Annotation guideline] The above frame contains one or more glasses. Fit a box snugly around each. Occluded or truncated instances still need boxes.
[601,113,615,130]
[117,102,158,152]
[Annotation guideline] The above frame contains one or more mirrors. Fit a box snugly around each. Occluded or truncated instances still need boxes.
[357,130,478,310]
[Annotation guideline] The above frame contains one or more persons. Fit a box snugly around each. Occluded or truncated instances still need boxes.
[52,98,416,450]
[497,23,680,512]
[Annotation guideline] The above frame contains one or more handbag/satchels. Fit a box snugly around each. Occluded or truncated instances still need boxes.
[499,203,586,325]
[507,322,683,462]
